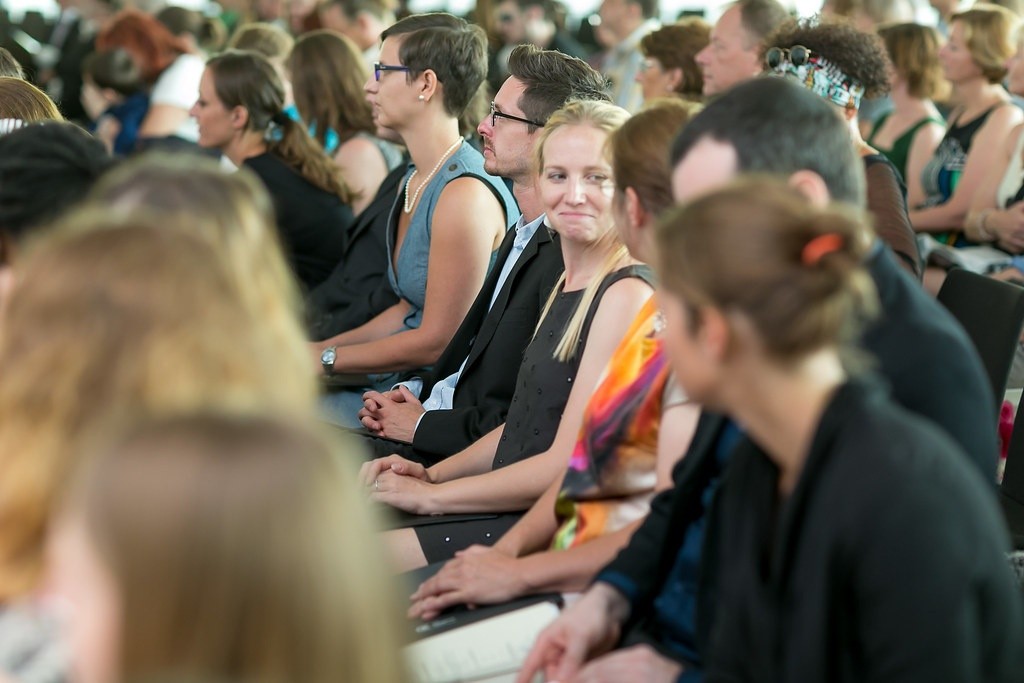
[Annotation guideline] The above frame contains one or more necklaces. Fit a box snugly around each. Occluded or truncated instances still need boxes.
[405,136,464,214]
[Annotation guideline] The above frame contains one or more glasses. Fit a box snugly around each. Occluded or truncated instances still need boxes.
[764,45,812,70]
[374,62,443,83]
[489,101,544,127]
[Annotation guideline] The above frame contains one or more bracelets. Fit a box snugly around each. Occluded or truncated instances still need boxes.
[979,209,996,241]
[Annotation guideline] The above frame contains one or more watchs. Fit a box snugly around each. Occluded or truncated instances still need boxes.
[321,345,338,376]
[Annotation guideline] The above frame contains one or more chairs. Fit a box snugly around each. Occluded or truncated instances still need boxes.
[935,267,1024,430]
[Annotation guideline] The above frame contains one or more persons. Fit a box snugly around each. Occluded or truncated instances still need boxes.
[655,176,1024,683]
[1,0,1024,683]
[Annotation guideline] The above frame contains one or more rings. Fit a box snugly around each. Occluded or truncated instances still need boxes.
[375,479,378,492]
